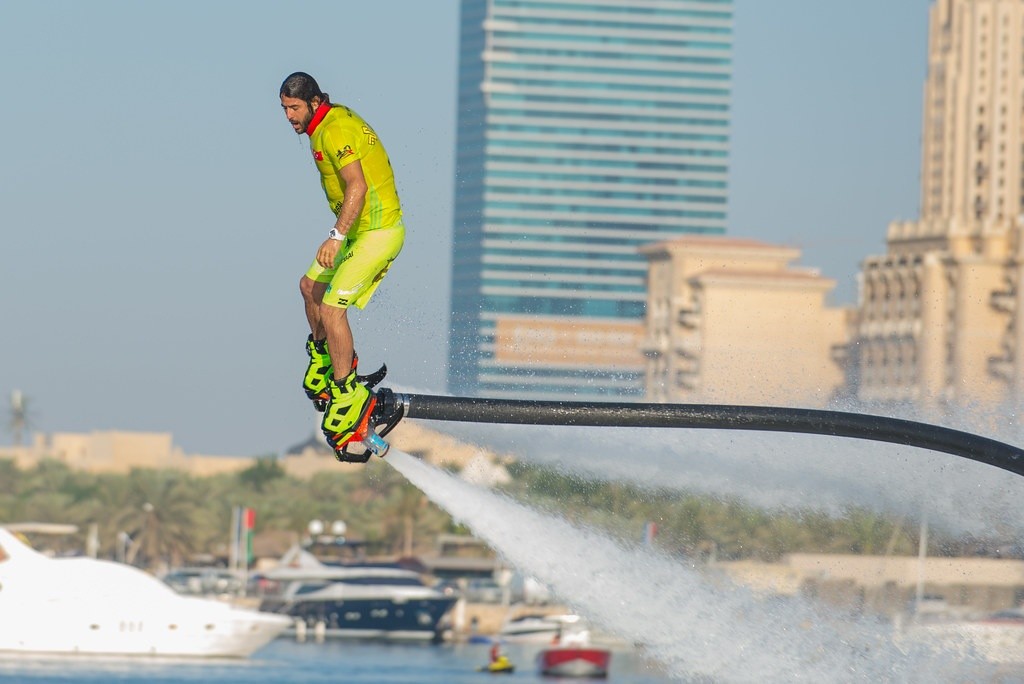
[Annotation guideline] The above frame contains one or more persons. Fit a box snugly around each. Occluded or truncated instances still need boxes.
[549,628,560,646]
[489,640,500,663]
[278,71,404,440]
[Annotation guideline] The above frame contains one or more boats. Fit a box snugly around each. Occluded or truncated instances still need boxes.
[490,656,514,674]
[0,525,296,657]
[502,614,559,643]
[540,624,610,679]
[256,520,460,641]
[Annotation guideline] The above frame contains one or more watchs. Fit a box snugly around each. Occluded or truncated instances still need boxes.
[328,228,346,241]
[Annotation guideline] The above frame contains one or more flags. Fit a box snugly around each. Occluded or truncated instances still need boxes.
[233,502,257,563]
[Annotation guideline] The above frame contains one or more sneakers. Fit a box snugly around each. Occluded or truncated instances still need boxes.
[300,334,359,397]
[326,377,378,449]
